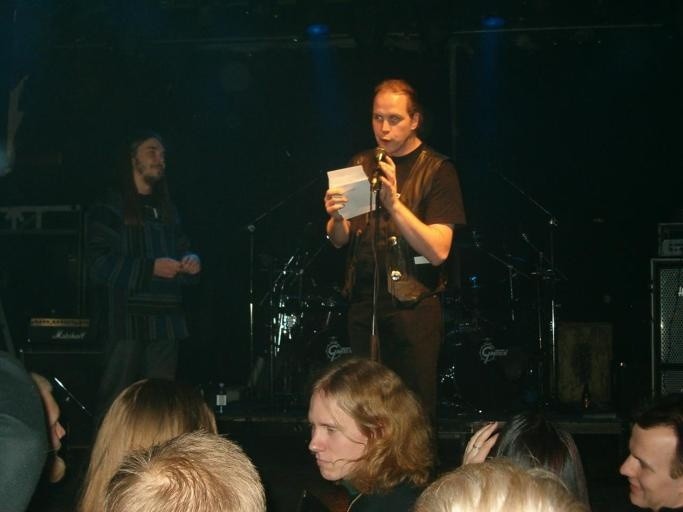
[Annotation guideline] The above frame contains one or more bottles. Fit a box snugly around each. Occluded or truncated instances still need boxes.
[215,383,227,416]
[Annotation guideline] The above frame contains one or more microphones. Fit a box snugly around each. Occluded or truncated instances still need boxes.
[370,148,385,190]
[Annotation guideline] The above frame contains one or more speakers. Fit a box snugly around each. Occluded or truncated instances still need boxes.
[645,257,682,402]
[1,203,106,344]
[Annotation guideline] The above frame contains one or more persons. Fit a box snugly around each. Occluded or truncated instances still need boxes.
[68,131,209,434]
[620,394,682,512]
[308,357,437,511]
[323,78,468,465]
[0,351,266,511]
[411,414,591,511]
